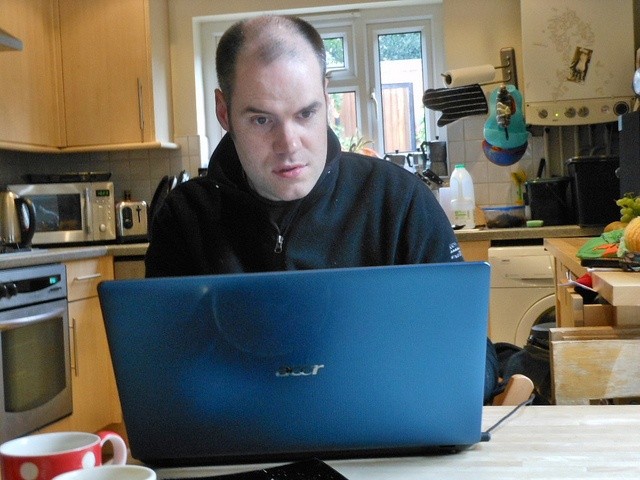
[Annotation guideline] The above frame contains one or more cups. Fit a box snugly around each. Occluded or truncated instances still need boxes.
[1,431,129,476]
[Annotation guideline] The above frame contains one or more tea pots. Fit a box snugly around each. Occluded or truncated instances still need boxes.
[407,147,421,168]
[382,150,408,171]
[422,137,447,175]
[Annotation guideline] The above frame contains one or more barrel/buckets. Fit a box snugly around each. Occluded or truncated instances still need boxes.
[449,163,476,231]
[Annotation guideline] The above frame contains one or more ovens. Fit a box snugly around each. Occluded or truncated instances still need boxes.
[1,264,72,440]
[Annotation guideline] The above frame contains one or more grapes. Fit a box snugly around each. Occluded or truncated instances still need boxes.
[615,196,640,223]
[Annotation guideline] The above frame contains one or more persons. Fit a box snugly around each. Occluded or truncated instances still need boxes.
[145,16,502,407]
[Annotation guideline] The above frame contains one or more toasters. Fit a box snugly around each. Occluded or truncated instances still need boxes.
[117,199,149,239]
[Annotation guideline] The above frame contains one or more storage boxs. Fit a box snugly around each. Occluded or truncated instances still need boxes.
[481,205,525,229]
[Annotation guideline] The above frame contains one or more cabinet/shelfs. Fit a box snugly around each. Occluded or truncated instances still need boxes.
[59,2,173,148]
[0,0,65,150]
[544,236,639,405]
[65,255,120,438]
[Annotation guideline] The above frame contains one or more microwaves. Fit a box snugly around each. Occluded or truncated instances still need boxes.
[4,181,116,244]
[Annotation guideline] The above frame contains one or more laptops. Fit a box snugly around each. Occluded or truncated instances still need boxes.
[96,261,491,468]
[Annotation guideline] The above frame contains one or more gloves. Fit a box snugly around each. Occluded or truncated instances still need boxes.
[424,84,488,127]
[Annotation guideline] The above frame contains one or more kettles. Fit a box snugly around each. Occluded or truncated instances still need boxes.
[1,188,35,252]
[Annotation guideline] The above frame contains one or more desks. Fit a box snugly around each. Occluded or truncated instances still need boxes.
[94,404,640,480]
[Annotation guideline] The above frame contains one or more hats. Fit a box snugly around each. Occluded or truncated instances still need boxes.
[482,85,527,165]
